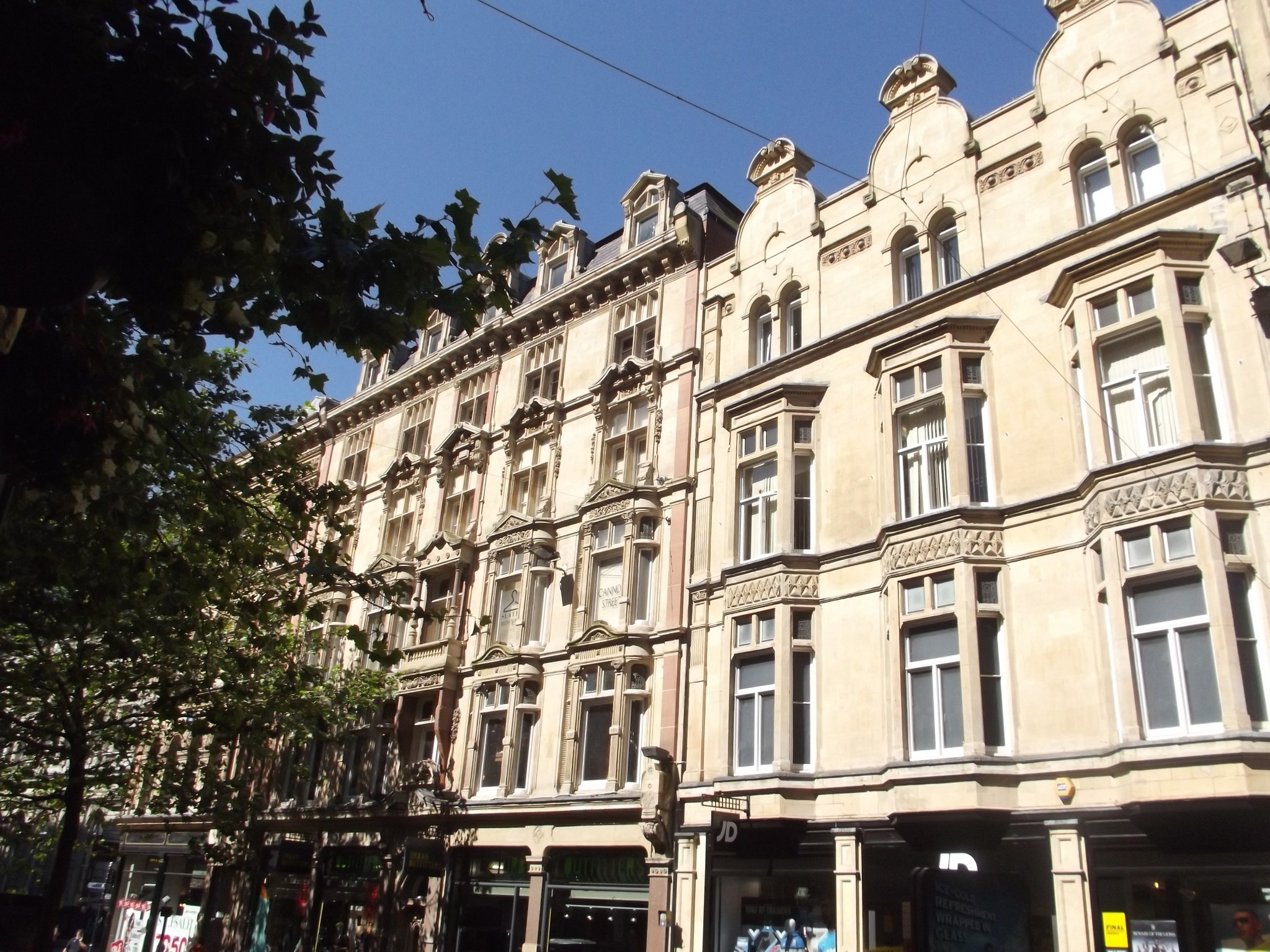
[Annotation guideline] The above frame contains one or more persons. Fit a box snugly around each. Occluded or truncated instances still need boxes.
[1232,908,1270,952]
[781,918,805,952]
[63,929,88,952]
[329,921,349,952]
[818,928,836,952]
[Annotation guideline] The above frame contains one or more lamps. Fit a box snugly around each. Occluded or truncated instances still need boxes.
[1214,237,1266,288]
[529,545,567,576]
